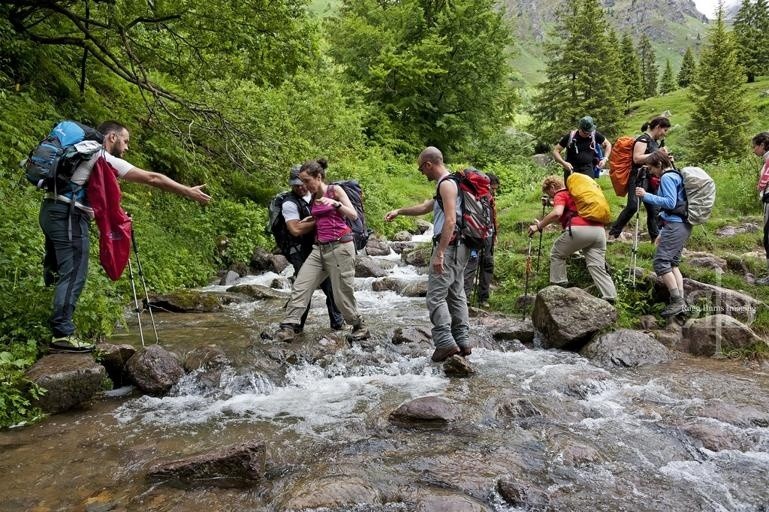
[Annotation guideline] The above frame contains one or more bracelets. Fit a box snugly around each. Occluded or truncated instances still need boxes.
[335,201,343,209]
[547,199,550,207]
[602,157,609,162]
[537,222,542,234]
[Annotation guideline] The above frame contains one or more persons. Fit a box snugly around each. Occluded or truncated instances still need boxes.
[279,163,346,334]
[751,133,768,283]
[553,117,611,190]
[277,158,371,341]
[607,117,674,243]
[464,174,498,308]
[37,120,213,352]
[529,175,617,310]
[383,146,473,359]
[636,153,698,316]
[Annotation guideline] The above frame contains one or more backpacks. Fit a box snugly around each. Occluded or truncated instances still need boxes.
[608,135,655,197]
[437,167,493,250]
[26,120,105,195]
[659,166,716,226]
[269,191,313,254]
[553,172,611,225]
[327,180,369,250]
[568,129,603,178]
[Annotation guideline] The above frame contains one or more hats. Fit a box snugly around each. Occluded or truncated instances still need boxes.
[580,116,597,131]
[288,165,303,186]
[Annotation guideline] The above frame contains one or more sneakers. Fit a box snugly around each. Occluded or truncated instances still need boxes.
[431,345,461,362]
[261,328,294,342]
[345,325,371,342]
[757,277,769,285]
[48,334,94,354]
[661,296,687,317]
[461,347,472,357]
